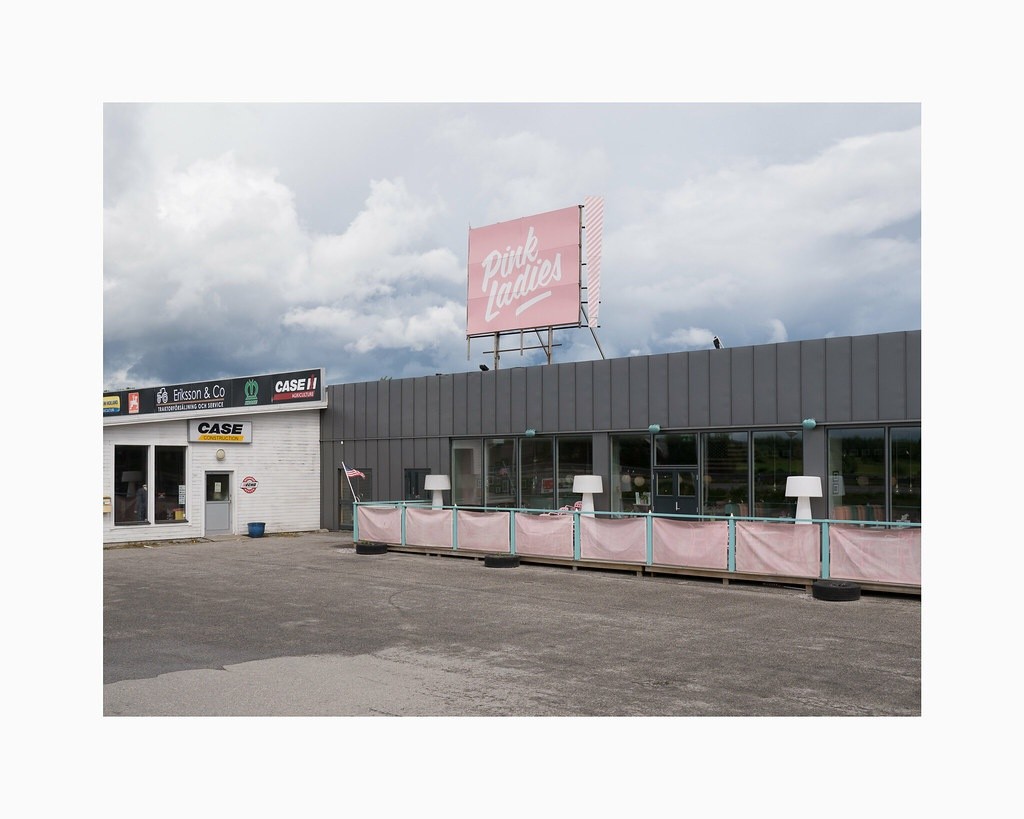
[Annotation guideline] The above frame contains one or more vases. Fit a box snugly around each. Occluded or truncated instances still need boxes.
[248,522,265,538]
[709,513,716,521]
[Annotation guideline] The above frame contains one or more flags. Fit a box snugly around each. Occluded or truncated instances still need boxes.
[344,463,365,478]
[500,463,507,475]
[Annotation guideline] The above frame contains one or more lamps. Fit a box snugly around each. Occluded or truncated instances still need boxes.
[713,336,725,349]
[215,449,226,462]
[649,424,661,435]
[479,365,489,371]
[525,429,536,437]
[803,419,817,430]
[622,472,646,487]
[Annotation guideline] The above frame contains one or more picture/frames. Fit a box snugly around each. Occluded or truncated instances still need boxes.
[488,466,528,496]
[655,471,698,498]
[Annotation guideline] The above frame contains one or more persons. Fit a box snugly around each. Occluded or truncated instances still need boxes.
[134,484,148,520]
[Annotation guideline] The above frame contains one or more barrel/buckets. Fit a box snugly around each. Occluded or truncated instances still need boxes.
[248,523,266,538]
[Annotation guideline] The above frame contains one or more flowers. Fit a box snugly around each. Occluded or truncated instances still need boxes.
[705,501,722,514]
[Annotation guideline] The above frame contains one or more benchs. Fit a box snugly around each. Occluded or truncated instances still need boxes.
[833,503,898,527]
[725,501,773,522]
